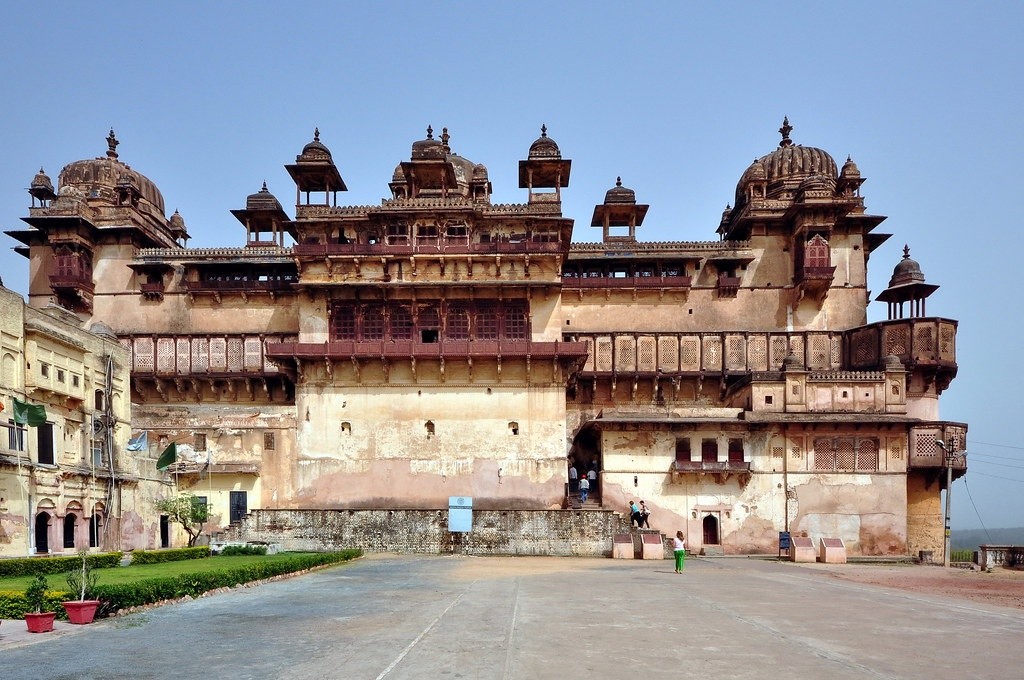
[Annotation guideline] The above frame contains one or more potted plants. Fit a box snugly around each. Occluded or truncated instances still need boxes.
[61,550,101,625]
[23,571,57,633]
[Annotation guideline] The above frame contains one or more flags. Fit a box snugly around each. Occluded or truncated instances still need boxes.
[127,431,147,452]
[12,395,47,427]
[155,442,177,470]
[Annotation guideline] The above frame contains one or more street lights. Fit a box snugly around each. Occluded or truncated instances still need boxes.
[936,439,968,567]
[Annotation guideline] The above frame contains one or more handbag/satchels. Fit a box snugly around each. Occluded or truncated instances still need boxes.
[643,507,651,515]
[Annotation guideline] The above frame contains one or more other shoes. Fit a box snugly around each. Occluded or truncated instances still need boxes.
[680,572,682,574]
[646,528,650,530]
[638,527,643,530]
[675,570,679,573]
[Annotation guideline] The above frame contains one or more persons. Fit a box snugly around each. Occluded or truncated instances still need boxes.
[629,501,642,529]
[587,467,597,493]
[639,501,651,530]
[569,465,578,491]
[592,452,599,471]
[670,531,687,574]
[579,474,589,503]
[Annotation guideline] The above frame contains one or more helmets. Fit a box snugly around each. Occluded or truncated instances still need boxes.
[582,475,586,477]
